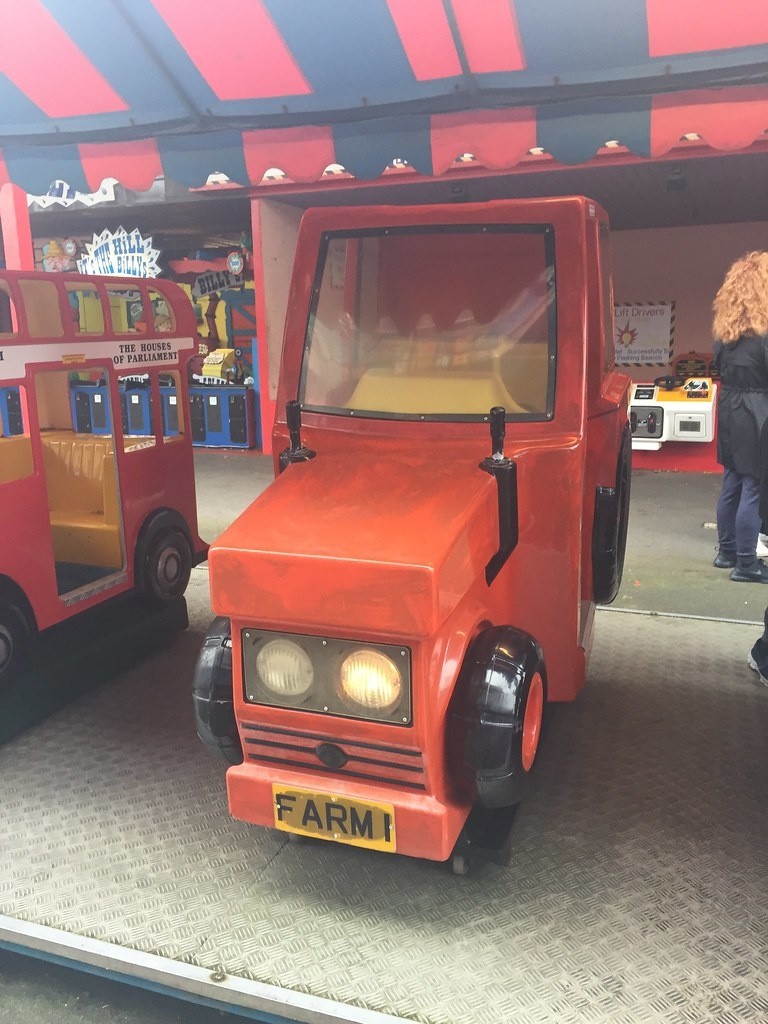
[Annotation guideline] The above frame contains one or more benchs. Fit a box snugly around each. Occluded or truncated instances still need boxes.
[0,432,184,571]
[328,334,549,413]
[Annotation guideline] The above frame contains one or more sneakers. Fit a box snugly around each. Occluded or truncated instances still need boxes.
[729,558,768,585]
[713,546,736,568]
[746,637,768,688]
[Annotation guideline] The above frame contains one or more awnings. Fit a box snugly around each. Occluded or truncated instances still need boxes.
[2,0,768,196]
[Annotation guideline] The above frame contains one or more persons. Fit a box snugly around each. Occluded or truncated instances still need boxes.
[747,607,768,687]
[713,252,768,584]
